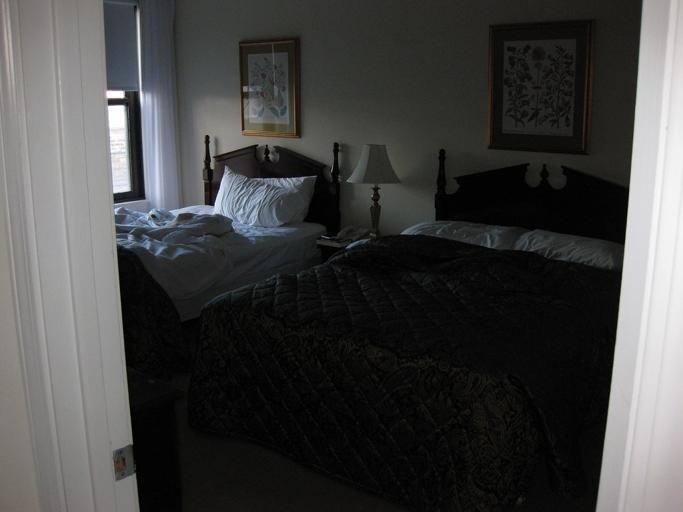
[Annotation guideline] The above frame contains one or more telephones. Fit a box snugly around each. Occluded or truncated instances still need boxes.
[337,225,369,242]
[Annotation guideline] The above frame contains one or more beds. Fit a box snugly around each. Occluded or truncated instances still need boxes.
[113,134,629,512]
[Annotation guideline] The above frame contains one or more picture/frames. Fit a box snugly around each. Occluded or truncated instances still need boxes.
[239,38,301,138]
[487,20,595,154]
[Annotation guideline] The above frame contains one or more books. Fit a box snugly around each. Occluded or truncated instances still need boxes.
[315,233,352,248]
